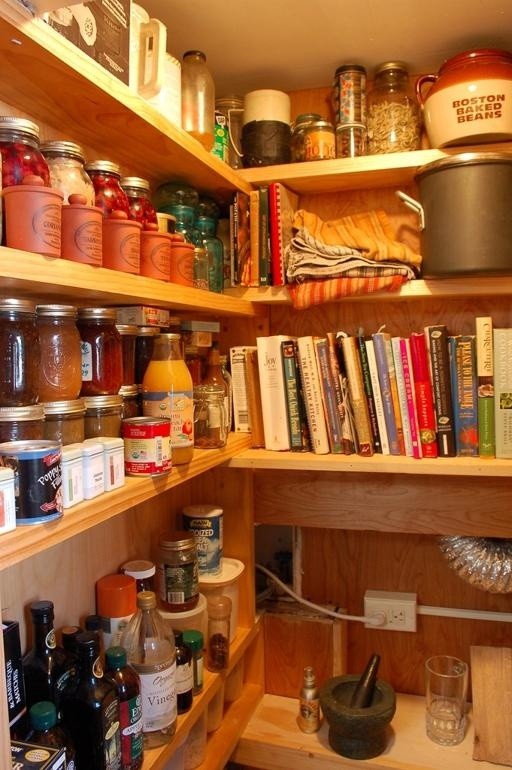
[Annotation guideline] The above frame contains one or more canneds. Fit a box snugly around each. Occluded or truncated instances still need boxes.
[0,439,64,527]
[121,414,174,478]
[180,502,224,574]
[154,530,201,612]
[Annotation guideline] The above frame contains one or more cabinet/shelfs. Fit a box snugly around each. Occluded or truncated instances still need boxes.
[1,3,511,770]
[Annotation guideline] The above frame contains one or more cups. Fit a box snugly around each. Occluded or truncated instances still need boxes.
[423,654,472,747]
[228,88,291,166]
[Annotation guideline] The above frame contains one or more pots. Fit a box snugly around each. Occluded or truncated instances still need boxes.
[396,148,512,277]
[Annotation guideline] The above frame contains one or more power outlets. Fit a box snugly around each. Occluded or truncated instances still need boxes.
[353,586,419,643]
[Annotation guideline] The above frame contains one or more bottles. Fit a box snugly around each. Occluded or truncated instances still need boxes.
[84,158,129,219]
[135,326,158,384]
[179,627,203,696]
[42,400,85,444]
[193,384,228,450]
[34,304,81,400]
[0,407,46,442]
[79,307,124,395]
[0,297,41,407]
[159,181,197,245]
[216,95,242,171]
[202,340,228,399]
[333,64,369,125]
[103,644,144,769]
[66,633,120,769]
[197,200,223,293]
[366,60,424,156]
[119,386,138,422]
[180,49,215,153]
[141,332,194,465]
[299,666,321,733]
[124,590,177,751]
[205,593,232,672]
[116,324,136,383]
[83,394,124,439]
[0,114,50,189]
[337,124,366,158]
[39,140,96,205]
[121,175,157,229]
[18,600,76,727]
[291,119,336,161]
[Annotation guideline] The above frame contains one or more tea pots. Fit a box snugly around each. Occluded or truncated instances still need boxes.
[415,48,512,149]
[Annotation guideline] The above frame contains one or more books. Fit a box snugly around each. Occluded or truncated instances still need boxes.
[225,183,304,287]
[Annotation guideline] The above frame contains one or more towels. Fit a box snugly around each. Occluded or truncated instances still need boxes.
[284,207,424,312]
